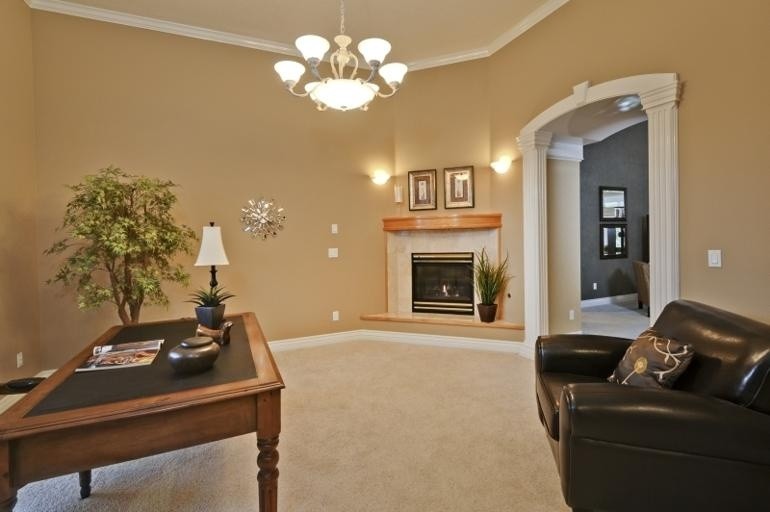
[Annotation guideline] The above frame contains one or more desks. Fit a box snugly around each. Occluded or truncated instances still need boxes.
[1,311,286,512]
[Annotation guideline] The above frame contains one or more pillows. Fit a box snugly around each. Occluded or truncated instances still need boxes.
[607,329,696,391]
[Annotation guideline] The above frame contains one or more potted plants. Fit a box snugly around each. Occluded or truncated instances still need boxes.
[183,285,236,329]
[462,247,517,323]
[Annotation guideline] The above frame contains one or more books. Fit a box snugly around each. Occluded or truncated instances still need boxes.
[75,339,165,373]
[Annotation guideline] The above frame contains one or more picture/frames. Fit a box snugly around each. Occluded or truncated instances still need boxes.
[407,169,437,212]
[443,165,476,210]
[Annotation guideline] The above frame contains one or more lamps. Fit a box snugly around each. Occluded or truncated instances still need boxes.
[192,222,231,288]
[273,0,410,113]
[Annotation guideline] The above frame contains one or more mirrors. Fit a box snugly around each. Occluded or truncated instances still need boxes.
[600,224,630,260]
[599,185,628,222]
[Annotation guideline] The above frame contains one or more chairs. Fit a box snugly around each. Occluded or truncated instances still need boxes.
[632,260,650,318]
[533,297,769,512]
